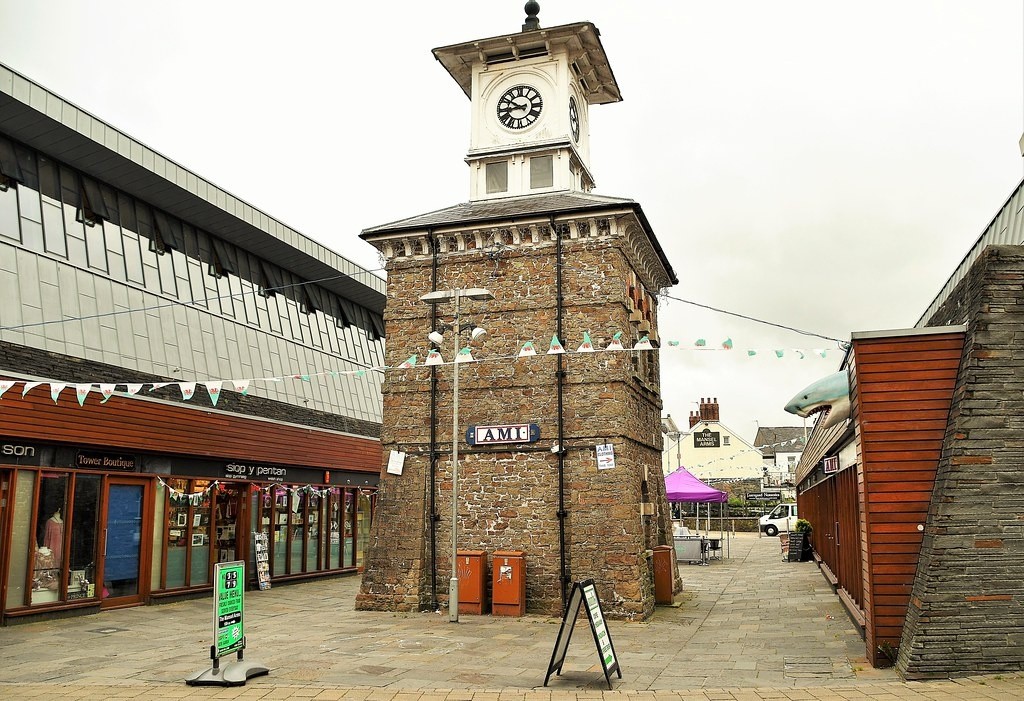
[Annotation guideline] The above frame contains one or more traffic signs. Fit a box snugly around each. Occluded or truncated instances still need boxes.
[746,492,763,501]
[763,492,780,500]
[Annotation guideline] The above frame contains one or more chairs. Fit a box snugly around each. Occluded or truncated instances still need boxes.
[689,539,721,565]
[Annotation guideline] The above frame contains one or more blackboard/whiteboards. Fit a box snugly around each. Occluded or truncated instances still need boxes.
[788,532,804,559]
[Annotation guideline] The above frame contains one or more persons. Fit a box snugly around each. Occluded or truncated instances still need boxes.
[44,511,63,568]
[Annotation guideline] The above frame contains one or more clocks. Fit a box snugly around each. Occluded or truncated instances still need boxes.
[496,85,543,130]
[569,97,580,143]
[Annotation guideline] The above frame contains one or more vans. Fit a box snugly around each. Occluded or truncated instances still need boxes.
[758,504,798,536]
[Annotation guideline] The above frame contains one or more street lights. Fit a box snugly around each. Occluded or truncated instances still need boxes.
[421,288,494,623]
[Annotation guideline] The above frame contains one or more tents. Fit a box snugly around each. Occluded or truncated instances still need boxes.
[664,466,729,566]
